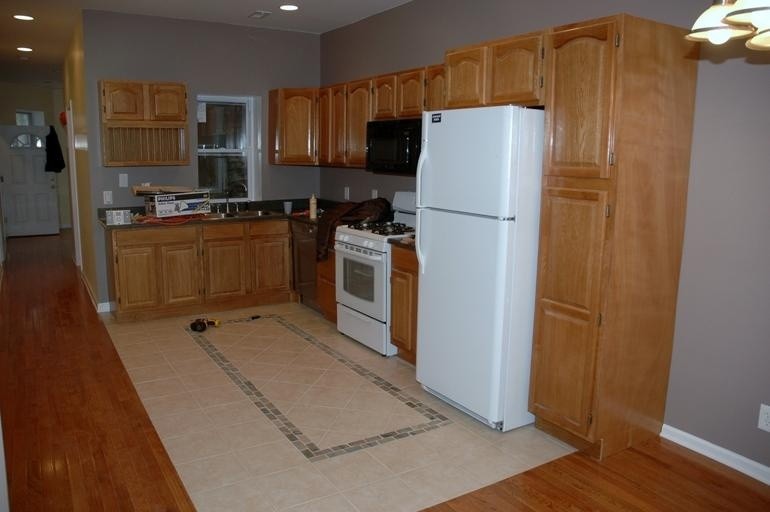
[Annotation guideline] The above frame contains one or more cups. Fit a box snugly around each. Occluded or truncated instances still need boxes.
[283,200,293,214]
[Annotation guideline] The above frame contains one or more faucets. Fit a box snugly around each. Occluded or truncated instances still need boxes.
[226,182,248,213]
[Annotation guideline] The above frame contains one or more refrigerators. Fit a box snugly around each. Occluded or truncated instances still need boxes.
[412,103,544,433]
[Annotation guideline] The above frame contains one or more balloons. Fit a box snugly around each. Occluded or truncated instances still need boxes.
[59,111,67,130]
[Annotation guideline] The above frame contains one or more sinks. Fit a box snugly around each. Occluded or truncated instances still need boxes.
[237,210,272,220]
[200,212,236,221]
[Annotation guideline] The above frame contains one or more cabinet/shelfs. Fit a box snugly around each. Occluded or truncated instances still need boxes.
[96,79,191,167]
[289,207,336,325]
[391,246,418,369]
[266,27,542,169]
[101,216,201,324]
[202,207,292,313]
[528,10,706,466]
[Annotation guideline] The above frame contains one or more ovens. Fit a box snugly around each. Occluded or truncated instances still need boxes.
[335,241,399,356]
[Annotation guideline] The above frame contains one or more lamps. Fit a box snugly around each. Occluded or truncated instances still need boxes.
[683,0,769,52]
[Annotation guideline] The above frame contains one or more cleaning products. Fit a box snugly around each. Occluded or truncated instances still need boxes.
[310,193,318,218]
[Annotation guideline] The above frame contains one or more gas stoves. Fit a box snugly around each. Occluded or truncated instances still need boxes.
[335,220,414,251]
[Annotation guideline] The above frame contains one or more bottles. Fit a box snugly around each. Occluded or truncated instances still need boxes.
[309,193,318,220]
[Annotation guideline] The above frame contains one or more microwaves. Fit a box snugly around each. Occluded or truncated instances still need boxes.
[364,117,421,176]
